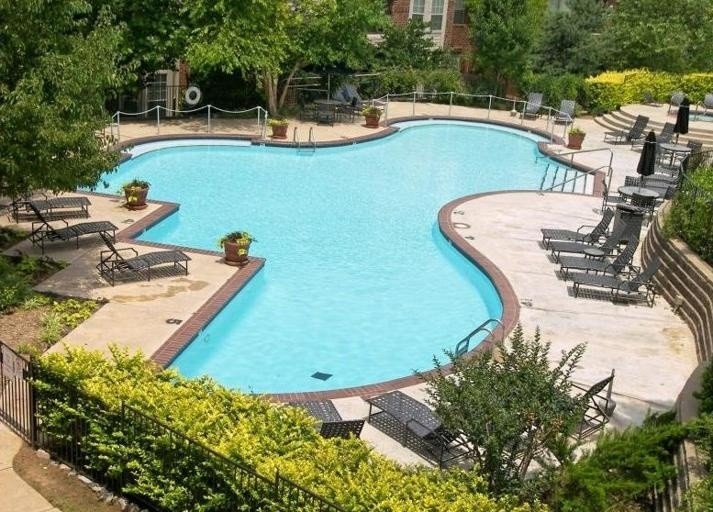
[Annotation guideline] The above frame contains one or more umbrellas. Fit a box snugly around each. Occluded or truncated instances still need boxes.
[299,61,352,100]
[635,128,656,192]
[667,95,689,166]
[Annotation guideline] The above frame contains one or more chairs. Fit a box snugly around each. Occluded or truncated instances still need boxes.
[519,92,577,125]
[604,114,703,208]
[289,390,480,469]
[300,95,318,121]
[555,373,614,446]
[7,190,192,287]
[539,204,663,309]
[342,97,357,123]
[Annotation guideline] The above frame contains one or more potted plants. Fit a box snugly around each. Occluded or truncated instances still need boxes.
[266,118,289,139]
[358,105,384,128]
[220,231,259,261]
[567,128,586,149]
[122,178,151,205]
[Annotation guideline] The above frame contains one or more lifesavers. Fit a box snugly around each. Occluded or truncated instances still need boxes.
[185,87,201,105]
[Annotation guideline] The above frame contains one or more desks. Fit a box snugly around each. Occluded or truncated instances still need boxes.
[312,99,342,128]
